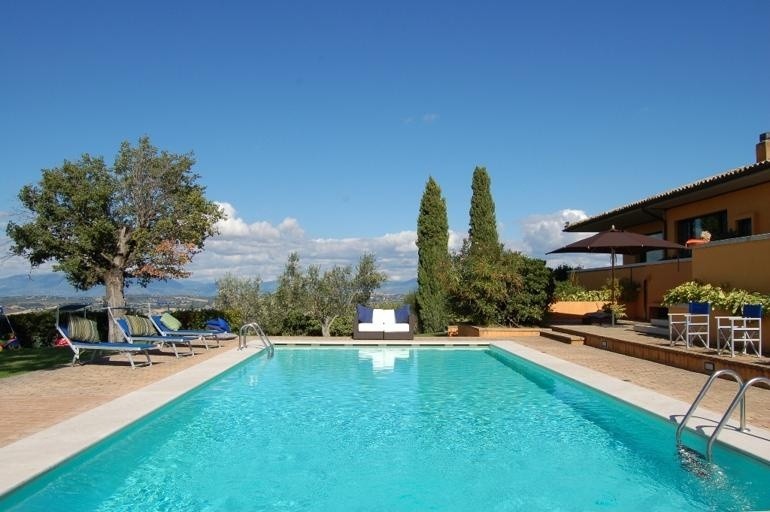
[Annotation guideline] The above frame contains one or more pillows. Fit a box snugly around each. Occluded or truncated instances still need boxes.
[373,308,383,324]
[160,312,183,331]
[384,309,396,324]
[357,303,373,323]
[67,312,101,344]
[394,303,412,324]
[124,311,156,337]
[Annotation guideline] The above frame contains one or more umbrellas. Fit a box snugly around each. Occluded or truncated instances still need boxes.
[544,223,691,329]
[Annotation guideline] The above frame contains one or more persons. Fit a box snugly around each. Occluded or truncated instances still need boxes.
[700,229,712,243]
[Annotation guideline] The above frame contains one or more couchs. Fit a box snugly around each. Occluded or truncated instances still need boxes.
[352,304,416,339]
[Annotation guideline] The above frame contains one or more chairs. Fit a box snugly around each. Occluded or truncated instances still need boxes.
[107,303,196,360]
[147,310,221,352]
[668,299,712,350]
[716,303,766,358]
[54,301,157,369]
